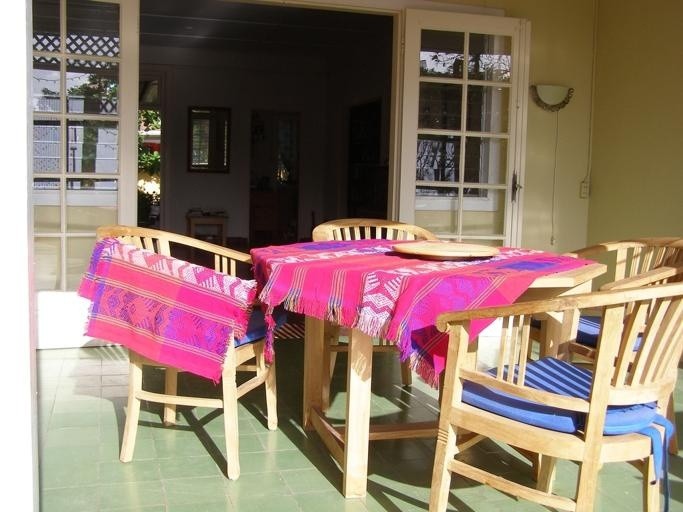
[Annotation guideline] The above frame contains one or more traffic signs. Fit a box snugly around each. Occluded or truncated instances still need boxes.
[580,181,589,198]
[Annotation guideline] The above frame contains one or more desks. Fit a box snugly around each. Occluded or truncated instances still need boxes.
[253,239,607,498]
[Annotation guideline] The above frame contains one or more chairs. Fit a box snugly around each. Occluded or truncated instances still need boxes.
[526,237,680,455]
[94,224,284,482]
[313,219,439,387]
[429,283,681,511]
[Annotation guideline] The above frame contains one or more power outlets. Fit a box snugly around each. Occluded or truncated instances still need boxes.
[580,181,589,198]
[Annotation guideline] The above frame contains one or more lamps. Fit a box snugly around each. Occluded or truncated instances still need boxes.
[94,224,284,482]
